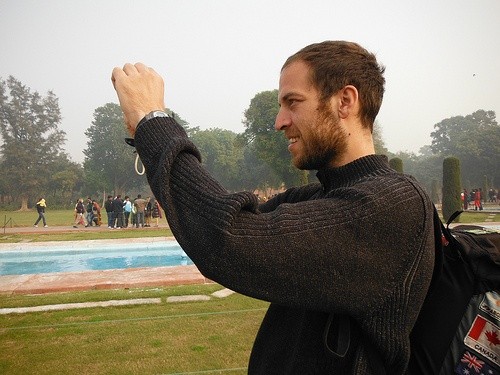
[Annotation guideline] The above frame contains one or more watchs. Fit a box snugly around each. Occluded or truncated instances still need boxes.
[125,110,170,147]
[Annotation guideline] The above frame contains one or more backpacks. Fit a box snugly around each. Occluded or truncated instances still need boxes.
[407,203,500,375]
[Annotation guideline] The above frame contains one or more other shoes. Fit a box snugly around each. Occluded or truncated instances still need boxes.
[108,225,158,229]
[43,225,48,227]
[72,223,102,228]
[33,224,38,227]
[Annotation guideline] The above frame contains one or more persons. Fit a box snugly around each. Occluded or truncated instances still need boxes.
[470,187,500,211]
[105,195,162,230]
[256,193,278,202]
[33,198,48,228]
[111,41,436,375]
[37,199,46,213]
[73,197,102,228]
